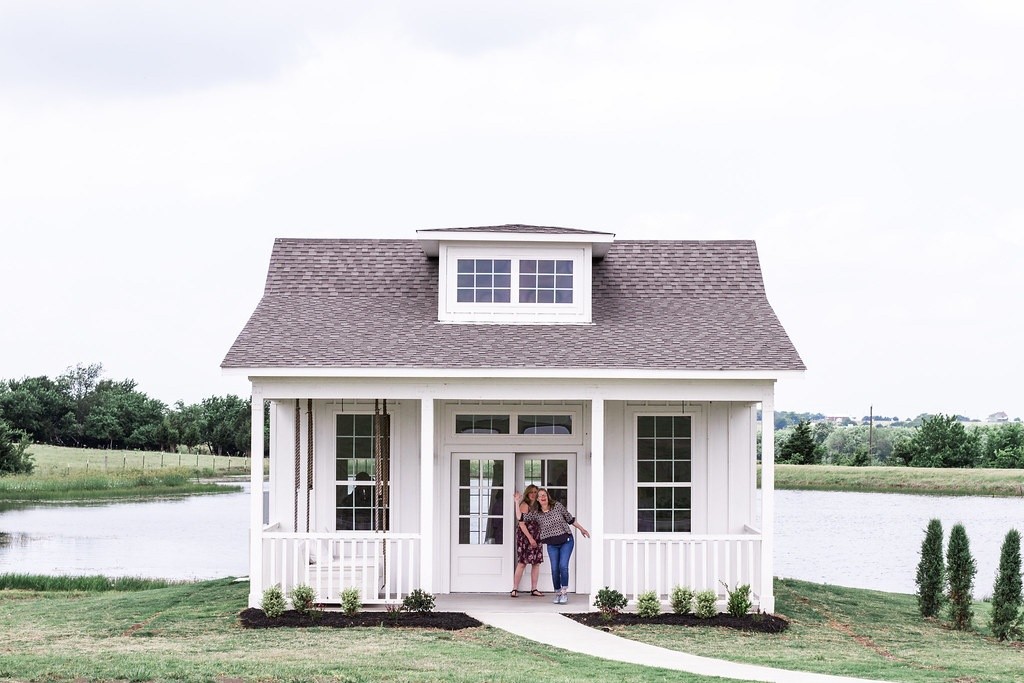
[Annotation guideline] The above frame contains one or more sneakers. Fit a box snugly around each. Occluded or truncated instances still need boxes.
[560,593,568,603]
[553,594,560,603]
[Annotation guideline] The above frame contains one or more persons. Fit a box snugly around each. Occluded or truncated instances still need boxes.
[490,483,503,544]
[511,484,544,597]
[515,488,590,604]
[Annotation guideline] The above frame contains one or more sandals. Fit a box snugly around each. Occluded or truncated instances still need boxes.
[531,589,545,596]
[510,590,518,597]
